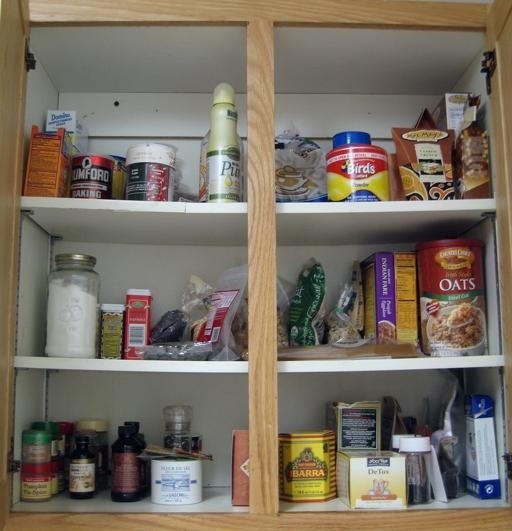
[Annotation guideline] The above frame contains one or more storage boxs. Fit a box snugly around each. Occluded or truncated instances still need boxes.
[228,425,250,507]
[44,108,85,197]
[21,127,79,196]
[336,447,412,514]
[355,250,415,347]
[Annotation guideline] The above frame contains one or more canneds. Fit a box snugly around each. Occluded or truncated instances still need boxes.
[71,153,115,199]
[325,144,391,202]
[414,238,488,359]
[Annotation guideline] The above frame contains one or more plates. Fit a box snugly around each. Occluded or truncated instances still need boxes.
[377,320,395,333]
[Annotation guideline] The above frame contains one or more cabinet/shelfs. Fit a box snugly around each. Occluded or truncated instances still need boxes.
[3,0,512,529]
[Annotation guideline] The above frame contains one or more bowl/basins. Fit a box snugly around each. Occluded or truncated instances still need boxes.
[427,305,486,357]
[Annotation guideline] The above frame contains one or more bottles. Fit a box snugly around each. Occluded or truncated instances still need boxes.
[390,433,431,506]
[162,405,192,455]
[43,253,100,358]
[23,419,147,499]
[204,82,243,203]
